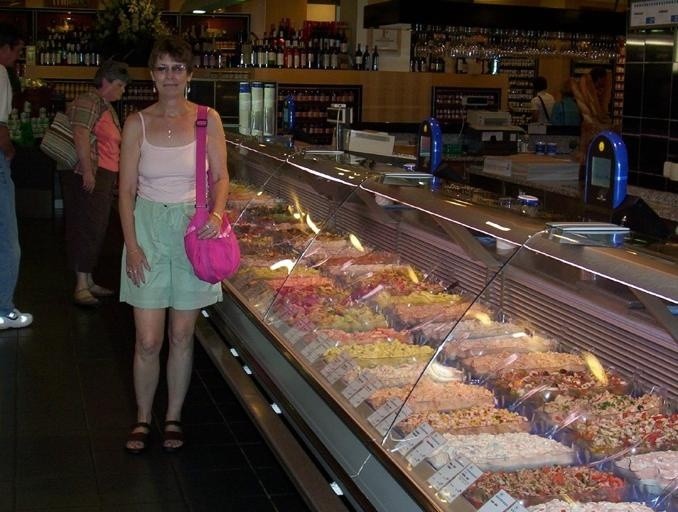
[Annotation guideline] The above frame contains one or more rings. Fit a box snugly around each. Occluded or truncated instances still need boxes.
[212,230,218,235]
[204,225,209,229]
[133,271,137,274]
[127,270,133,273]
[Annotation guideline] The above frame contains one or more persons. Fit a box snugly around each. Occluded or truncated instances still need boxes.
[118,33,239,453]
[549,80,582,137]
[42,55,129,305]
[530,76,556,136]
[592,68,607,103]
[0,27,33,331]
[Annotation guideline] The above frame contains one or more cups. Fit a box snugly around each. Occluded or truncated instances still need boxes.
[547,143,558,156]
[536,142,546,156]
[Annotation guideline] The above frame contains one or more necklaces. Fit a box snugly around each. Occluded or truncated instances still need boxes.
[166,129,176,141]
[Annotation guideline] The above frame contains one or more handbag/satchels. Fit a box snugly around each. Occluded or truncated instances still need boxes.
[40,111,97,172]
[184,201,241,285]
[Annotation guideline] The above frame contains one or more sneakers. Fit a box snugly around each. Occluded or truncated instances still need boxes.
[0,307,34,330]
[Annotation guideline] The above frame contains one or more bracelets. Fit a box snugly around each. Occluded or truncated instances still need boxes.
[210,208,225,223]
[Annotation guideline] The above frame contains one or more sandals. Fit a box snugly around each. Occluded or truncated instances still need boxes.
[125,416,155,457]
[88,280,114,299]
[72,289,100,308]
[158,419,185,457]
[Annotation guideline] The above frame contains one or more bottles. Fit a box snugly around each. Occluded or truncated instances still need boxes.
[278,31,286,51]
[433,87,496,131]
[257,40,265,67]
[265,40,270,67]
[95,44,100,66]
[119,86,158,122]
[66,43,71,64]
[208,50,215,68]
[277,39,288,67]
[411,23,616,73]
[313,27,319,49]
[201,51,208,65]
[331,43,338,68]
[56,41,63,65]
[355,43,363,70]
[319,28,324,49]
[329,28,335,49]
[45,40,50,65]
[284,40,294,68]
[38,103,64,118]
[51,40,56,65]
[292,40,300,68]
[38,41,44,65]
[341,28,349,53]
[84,45,90,67]
[91,45,95,64]
[335,28,341,53]
[236,31,244,67]
[76,44,80,65]
[251,40,257,66]
[322,43,330,69]
[363,45,371,70]
[215,49,222,69]
[278,88,355,137]
[315,45,322,68]
[47,23,92,42]
[268,39,278,66]
[307,41,316,69]
[372,45,379,71]
[79,45,84,65]
[322,28,329,51]
[71,43,77,65]
[271,31,278,50]
[299,39,307,69]
[508,73,536,122]
[52,84,94,100]
[61,42,67,65]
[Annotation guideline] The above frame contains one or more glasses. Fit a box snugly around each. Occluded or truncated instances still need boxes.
[153,64,184,76]
